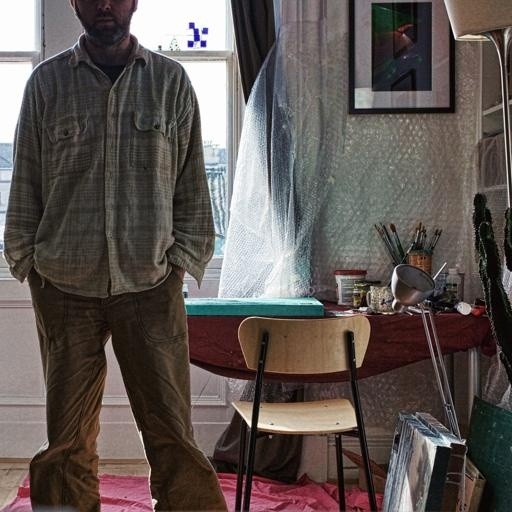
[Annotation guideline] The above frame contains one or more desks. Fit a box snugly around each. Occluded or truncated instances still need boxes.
[185,295,491,435]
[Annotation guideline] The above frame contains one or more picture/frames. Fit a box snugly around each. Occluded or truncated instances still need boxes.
[348,1,456,115]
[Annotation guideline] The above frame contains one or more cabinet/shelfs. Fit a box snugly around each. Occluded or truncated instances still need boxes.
[475,41,512,262]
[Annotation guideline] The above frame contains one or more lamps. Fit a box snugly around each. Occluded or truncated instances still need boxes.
[389,263,467,441]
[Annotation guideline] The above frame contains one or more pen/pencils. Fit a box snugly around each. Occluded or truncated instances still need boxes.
[433,262,448,281]
[405,310,414,316]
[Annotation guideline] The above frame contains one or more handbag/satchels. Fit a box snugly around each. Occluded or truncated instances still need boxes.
[216,380,304,480]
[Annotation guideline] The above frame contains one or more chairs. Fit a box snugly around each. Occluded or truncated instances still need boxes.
[231,316,378,511]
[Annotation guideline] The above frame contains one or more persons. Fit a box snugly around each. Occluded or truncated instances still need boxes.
[4,0,227,512]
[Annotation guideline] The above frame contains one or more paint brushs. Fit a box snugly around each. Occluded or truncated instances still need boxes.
[387,241,415,288]
[374,222,442,268]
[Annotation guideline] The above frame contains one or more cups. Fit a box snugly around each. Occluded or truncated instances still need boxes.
[366,284,392,314]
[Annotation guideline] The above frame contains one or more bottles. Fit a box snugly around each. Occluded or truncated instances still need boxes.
[445,267,464,308]
